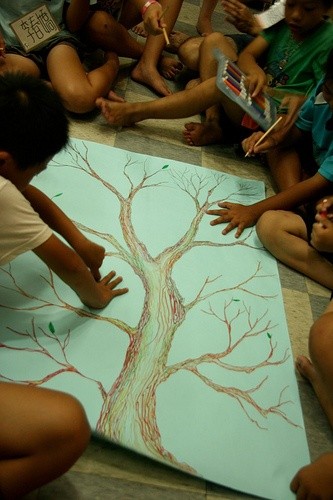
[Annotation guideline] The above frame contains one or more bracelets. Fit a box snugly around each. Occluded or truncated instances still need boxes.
[140,0,157,18]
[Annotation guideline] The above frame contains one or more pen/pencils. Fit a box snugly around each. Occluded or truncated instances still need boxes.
[222,58,272,121]
[157,5,171,46]
[242,116,283,159]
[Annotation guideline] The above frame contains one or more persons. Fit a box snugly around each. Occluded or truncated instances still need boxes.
[0,0,333,500]
